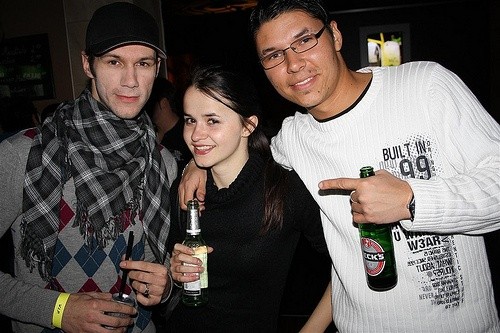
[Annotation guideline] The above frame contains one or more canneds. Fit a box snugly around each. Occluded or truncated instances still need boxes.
[103,292,135,330]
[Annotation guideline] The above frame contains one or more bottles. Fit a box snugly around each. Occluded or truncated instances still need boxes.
[181,199,210,307]
[357,166,398,292]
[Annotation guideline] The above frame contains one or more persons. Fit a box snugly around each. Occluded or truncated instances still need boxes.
[0,0,500,333]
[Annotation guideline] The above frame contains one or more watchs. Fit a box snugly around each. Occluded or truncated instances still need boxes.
[407,194,415,221]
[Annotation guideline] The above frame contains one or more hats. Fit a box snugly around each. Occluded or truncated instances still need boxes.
[85,2,168,60]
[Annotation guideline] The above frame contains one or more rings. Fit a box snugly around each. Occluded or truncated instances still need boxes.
[141,284,150,295]
[349,190,358,203]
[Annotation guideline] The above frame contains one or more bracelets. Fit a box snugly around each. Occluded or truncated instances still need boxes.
[182,160,194,177]
[52,292,71,328]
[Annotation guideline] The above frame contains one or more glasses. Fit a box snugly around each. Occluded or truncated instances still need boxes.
[260,22,330,70]
